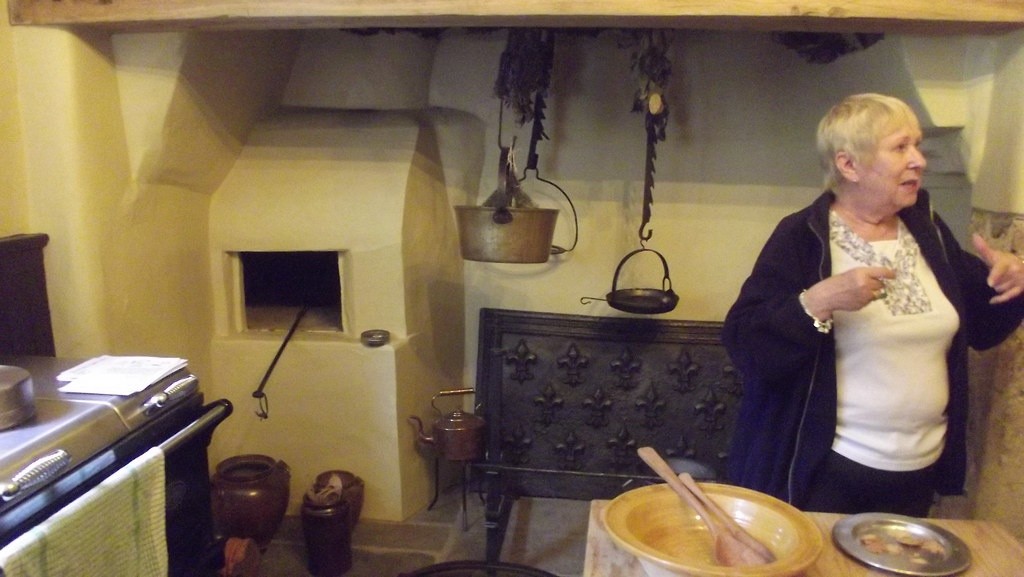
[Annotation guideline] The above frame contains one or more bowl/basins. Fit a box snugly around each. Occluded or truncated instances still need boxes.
[602,482,824,577]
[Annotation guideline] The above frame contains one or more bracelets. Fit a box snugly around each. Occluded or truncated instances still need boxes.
[799,290,833,333]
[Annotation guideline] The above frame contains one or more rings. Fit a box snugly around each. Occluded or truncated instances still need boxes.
[880,288,887,299]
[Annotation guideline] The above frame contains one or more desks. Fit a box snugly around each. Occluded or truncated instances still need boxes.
[583,500,1024,577]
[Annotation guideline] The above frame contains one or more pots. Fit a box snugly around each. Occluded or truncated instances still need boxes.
[453,147,560,265]
[606,248,679,315]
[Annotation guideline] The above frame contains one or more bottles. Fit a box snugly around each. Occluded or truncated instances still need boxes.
[210,455,294,551]
[301,468,364,574]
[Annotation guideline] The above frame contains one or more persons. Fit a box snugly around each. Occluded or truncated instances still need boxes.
[714,93,1024,518]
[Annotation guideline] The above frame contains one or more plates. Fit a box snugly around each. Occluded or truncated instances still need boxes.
[832,511,972,576]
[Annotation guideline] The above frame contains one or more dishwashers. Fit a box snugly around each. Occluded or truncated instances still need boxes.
[0,392,235,577]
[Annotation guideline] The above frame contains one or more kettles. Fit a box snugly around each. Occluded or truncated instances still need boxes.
[408,388,489,459]
